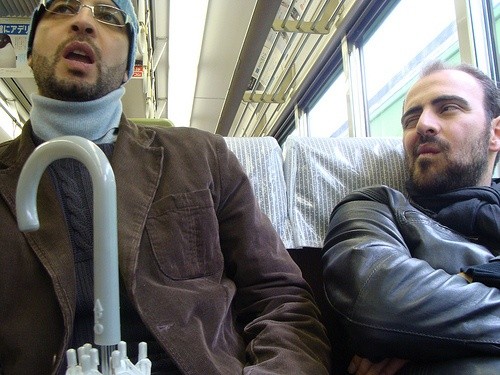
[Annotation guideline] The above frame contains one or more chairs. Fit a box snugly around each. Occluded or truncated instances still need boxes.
[216,135,298,266]
[284,134,412,312]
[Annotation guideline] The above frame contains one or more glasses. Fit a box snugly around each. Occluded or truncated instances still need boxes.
[37,0,132,36]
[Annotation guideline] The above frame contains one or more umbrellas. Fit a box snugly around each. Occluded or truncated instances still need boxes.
[15,136,152,375]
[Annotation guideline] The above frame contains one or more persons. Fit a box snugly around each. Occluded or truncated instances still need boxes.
[0,0,331,375]
[321,61,500,375]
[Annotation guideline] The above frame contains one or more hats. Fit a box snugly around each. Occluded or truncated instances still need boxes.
[26,0,138,86]
